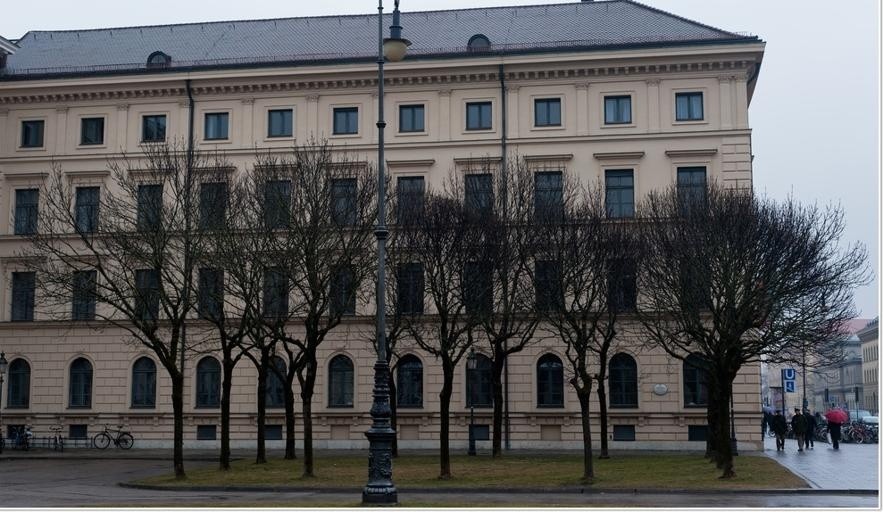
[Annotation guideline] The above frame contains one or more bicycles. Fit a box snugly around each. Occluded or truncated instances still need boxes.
[0,425,30,453]
[49,424,64,453]
[766,418,878,445]
[93,423,133,451]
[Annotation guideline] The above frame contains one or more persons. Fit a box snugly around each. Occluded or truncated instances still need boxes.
[760,407,840,453]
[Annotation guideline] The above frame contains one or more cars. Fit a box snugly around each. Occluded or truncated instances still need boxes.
[852,416,877,433]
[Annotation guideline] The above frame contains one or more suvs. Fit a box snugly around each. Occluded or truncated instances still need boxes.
[847,411,871,421]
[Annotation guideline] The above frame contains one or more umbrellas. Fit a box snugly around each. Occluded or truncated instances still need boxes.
[823,410,847,423]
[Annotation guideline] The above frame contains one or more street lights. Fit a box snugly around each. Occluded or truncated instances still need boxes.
[465,347,478,454]
[360,0,411,506]
